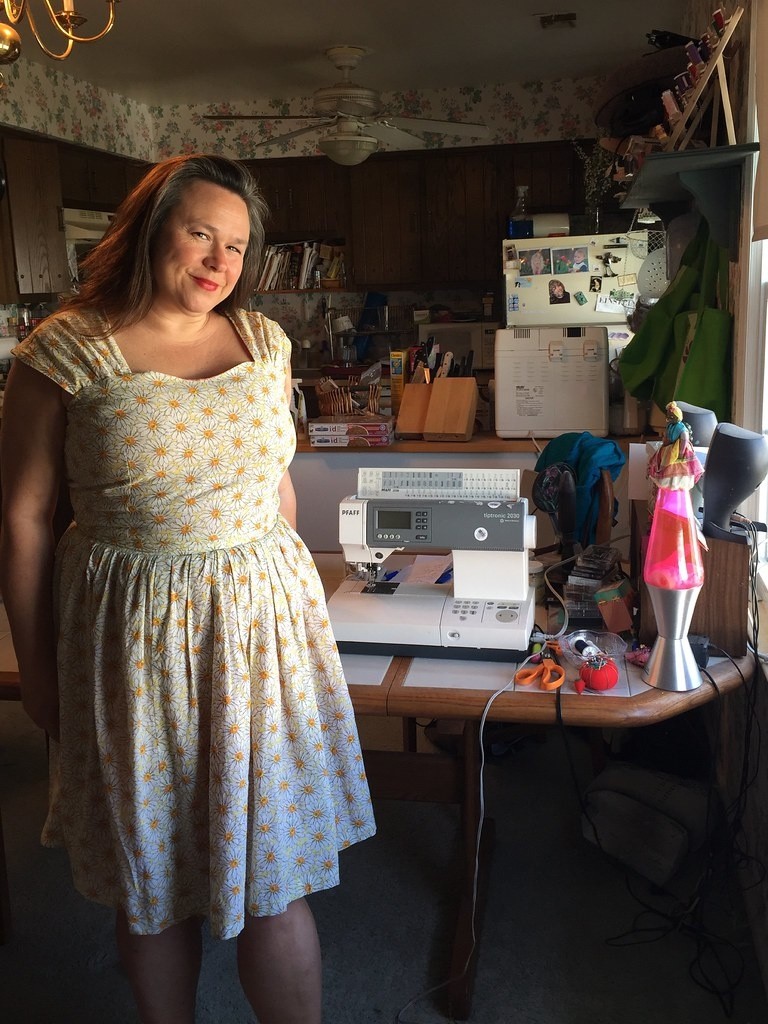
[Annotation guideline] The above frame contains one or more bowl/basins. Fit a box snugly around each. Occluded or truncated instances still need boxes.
[560,630,628,669]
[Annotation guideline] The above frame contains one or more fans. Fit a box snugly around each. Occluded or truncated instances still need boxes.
[202,45,490,149]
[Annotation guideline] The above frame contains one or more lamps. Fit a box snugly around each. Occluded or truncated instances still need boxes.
[0,0,117,70]
[318,123,379,166]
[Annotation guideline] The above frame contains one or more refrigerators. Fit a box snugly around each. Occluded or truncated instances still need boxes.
[503,232,649,363]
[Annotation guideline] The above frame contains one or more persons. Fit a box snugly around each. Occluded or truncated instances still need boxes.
[506,245,516,261]
[548,279,570,304]
[589,278,601,292]
[530,249,545,275]
[4,149,387,1024]
[515,282,520,287]
[570,248,589,272]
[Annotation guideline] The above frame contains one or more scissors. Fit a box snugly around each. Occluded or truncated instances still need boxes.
[513,645,566,691]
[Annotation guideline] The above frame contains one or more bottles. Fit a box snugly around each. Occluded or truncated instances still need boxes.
[298,340,312,368]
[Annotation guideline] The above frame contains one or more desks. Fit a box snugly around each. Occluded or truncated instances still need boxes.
[1,548,755,1019]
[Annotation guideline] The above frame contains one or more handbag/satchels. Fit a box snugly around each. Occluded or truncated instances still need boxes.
[618,217,735,424]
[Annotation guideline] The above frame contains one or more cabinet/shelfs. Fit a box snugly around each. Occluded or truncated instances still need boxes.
[0,121,601,304]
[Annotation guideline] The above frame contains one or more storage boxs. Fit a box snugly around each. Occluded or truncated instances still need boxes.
[308,416,395,447]
[413,310,431,324]
[390,349,411,417]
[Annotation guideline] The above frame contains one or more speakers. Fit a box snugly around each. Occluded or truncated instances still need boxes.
[677,400,718,447]
[701,422,768,544]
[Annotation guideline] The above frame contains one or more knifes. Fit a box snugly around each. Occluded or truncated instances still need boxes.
[411,336,475,384]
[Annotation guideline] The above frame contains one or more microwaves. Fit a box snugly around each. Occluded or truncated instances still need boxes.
[418,321,500,370]
